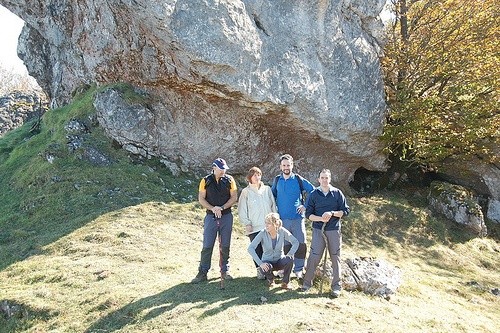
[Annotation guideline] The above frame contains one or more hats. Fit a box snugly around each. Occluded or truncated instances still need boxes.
[213,158,229,170]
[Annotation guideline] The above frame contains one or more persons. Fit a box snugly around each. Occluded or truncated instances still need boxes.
[247,212,299,288]
[271,153,316,279]
[192,157,237,283]
[237,166,278,279]
[300,168,350,296]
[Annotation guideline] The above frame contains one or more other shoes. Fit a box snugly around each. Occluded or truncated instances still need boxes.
[295,271,303,280]
[331,290,340,297]
[266,273,275,286]
[281,281,287,288]
[191,270,207,284]
[300,284,310,292]
[221,271,233,280]
[257,267,265,279]
[277,272,284,278]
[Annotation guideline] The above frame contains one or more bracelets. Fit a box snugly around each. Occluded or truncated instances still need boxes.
[222,206,224,210]
[331,211,334,215]
